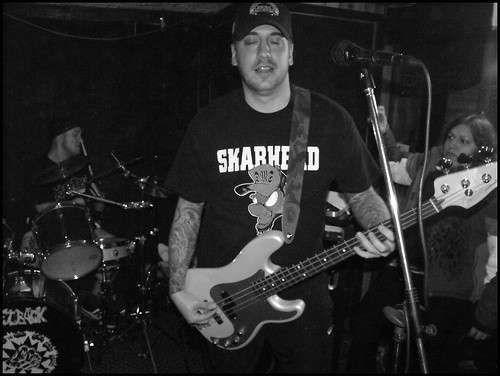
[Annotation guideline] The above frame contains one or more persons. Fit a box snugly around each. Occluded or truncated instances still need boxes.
[164,2,396,374]
[367,105,499,373]
[27,115,146,373]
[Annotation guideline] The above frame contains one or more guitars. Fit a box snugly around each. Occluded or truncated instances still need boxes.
[179,142,498,353]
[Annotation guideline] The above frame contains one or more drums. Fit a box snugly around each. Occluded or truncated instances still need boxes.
[325,221,348,294]
[2,268,83,373]
[89,263,125,300]
[98,231,141,272]
[31,204,105,279]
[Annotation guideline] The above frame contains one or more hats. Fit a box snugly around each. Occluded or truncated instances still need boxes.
[232,1,292,42]
[48,117,81,138]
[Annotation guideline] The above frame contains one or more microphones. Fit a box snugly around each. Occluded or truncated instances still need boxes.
[331,40,422,66]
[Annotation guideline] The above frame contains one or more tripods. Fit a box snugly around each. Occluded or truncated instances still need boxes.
[94,167,176,373]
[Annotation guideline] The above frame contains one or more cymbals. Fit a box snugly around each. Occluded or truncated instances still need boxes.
[36,154,98,184]
[87,153,148,183]
